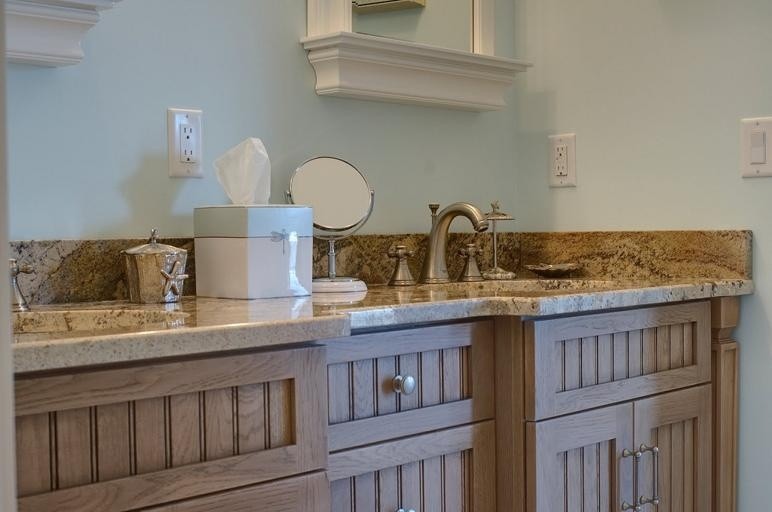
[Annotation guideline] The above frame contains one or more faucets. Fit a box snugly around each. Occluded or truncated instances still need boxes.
[419,201,490,283]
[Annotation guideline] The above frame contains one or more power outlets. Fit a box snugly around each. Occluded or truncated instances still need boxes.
[547,135,577,187]
[166,107,205,179]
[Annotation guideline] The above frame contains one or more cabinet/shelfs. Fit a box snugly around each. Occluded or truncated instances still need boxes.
[17,342,331,512]
[497,298,740,512]
[316,314,498,512]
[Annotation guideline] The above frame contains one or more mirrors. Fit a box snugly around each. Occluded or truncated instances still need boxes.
[286,156,373,293]
[301,0,533,112]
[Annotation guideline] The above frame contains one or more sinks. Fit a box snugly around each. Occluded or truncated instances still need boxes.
[415,279,618,294]
[9,311,192,334]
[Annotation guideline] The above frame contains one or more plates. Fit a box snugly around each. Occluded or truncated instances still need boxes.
[524,262,577,277]
